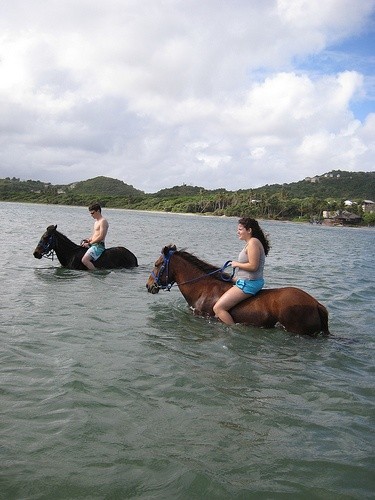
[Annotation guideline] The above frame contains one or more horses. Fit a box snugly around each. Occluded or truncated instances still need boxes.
[146,243,335,337]
[33,224,139,270]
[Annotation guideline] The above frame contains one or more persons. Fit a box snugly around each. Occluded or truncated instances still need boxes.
[80,204,109,271]
[213,217,273,327]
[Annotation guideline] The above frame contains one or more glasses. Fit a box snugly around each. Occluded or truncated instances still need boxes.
[90,212,96,214]
